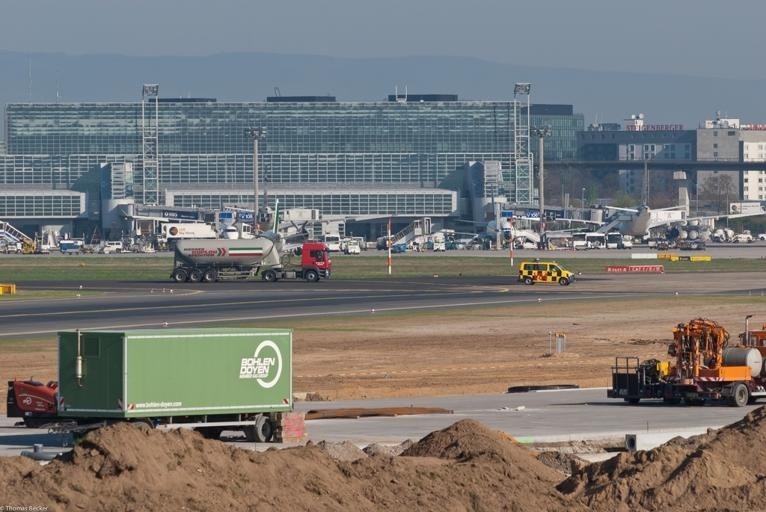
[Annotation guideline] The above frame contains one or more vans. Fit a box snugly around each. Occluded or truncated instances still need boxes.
[104,240,122,252]
[518,258,574,285]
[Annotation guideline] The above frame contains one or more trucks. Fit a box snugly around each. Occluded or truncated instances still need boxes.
[169,239,332,283]
[607,314,766,407]
[160,221,255,250]
[324,234,386,254]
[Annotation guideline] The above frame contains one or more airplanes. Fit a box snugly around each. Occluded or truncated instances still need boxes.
[518,204,764,237]
